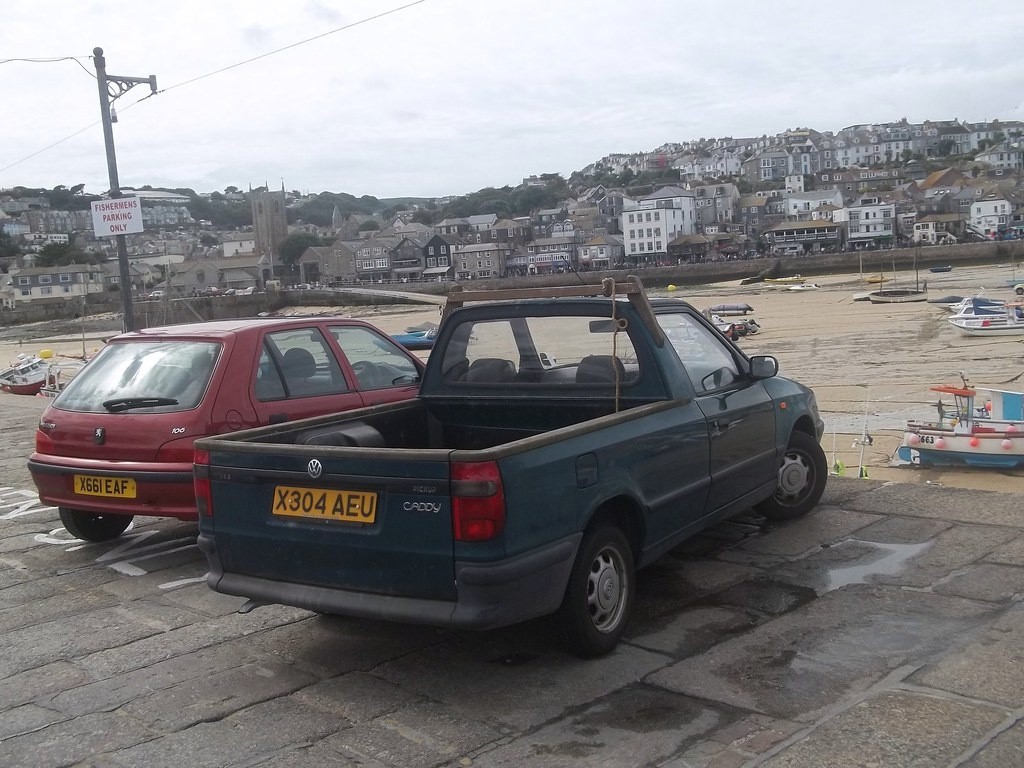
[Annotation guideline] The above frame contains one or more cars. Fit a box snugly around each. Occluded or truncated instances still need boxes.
[149,287,224,300]
[28,318,426,542]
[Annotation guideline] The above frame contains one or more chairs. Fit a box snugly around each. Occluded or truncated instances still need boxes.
[575,355,627,383]
[469,358,515,382]
[281,348,330,393]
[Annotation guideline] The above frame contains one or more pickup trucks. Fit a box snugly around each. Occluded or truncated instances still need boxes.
[193,276,827,649]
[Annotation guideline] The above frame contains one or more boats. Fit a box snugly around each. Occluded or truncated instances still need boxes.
[898,372,1024,468]
[740,271,822,291]
[0,353,86,398]
[852,265,1024,336]
[701,304,761,341]
